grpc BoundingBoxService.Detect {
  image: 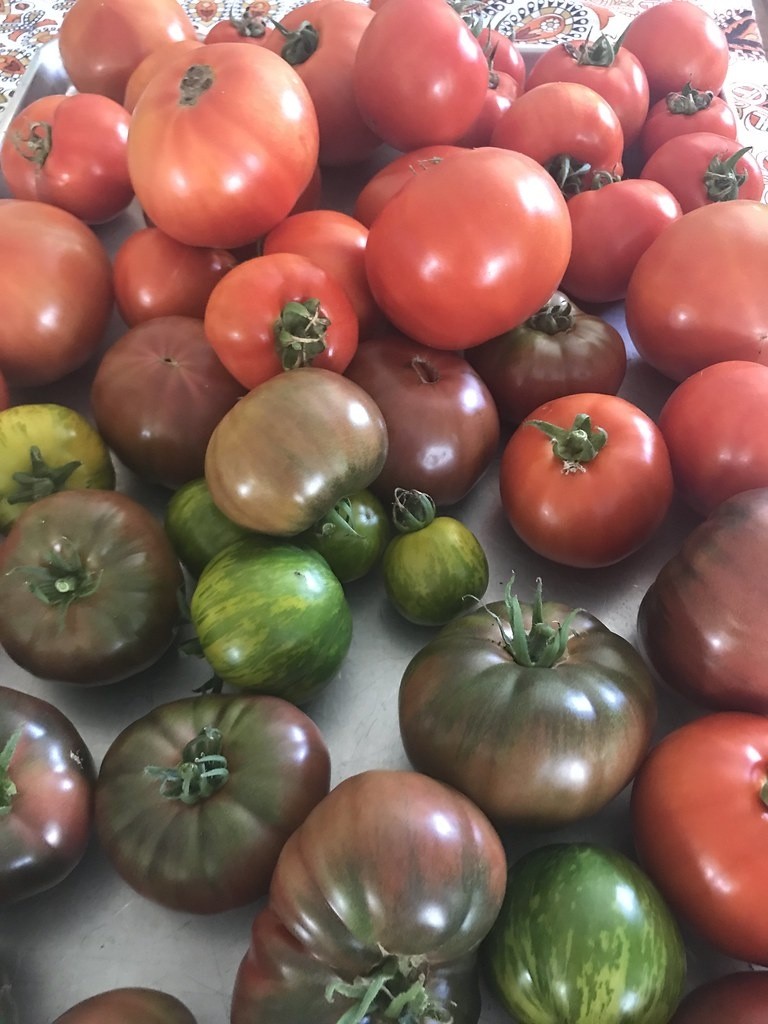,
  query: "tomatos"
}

[0,0,768,1024]
